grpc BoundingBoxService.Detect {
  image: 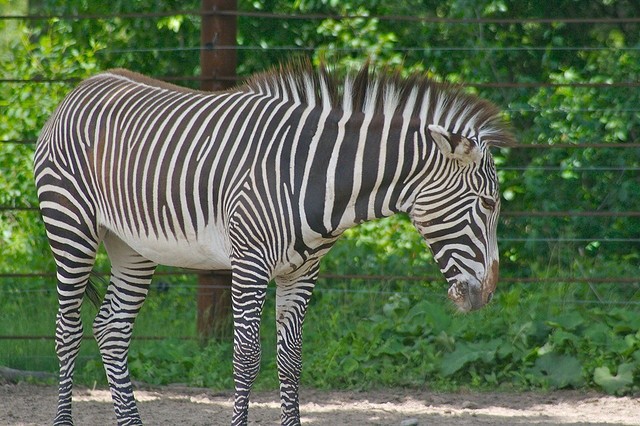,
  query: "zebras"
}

[34,56,519,426]
